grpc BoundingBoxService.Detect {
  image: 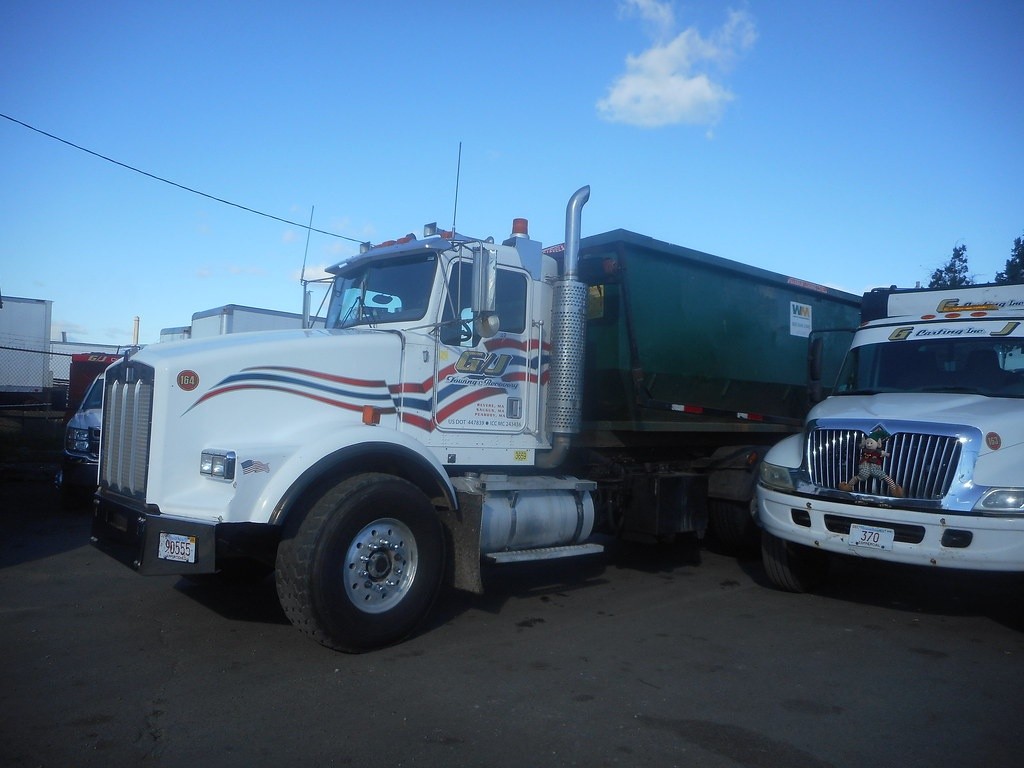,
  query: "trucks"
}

[61,372,128,491]
[94,184,865,656]
[741,282,1024,619]
